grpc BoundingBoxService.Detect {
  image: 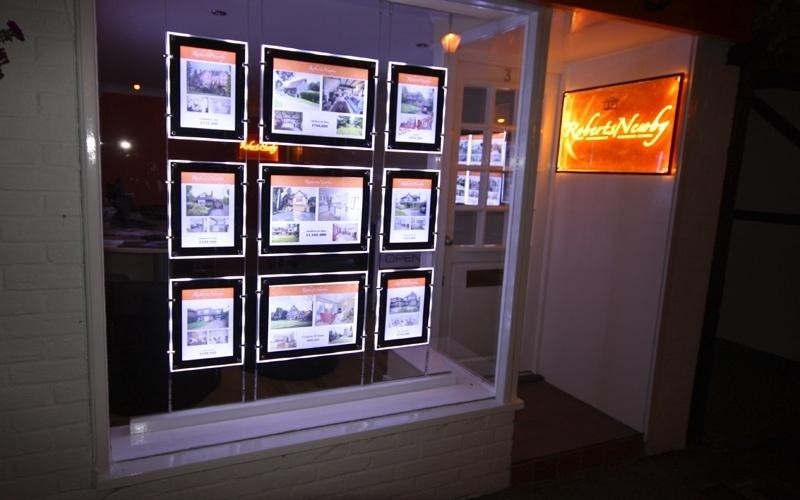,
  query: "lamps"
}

[441,12,461,53]
